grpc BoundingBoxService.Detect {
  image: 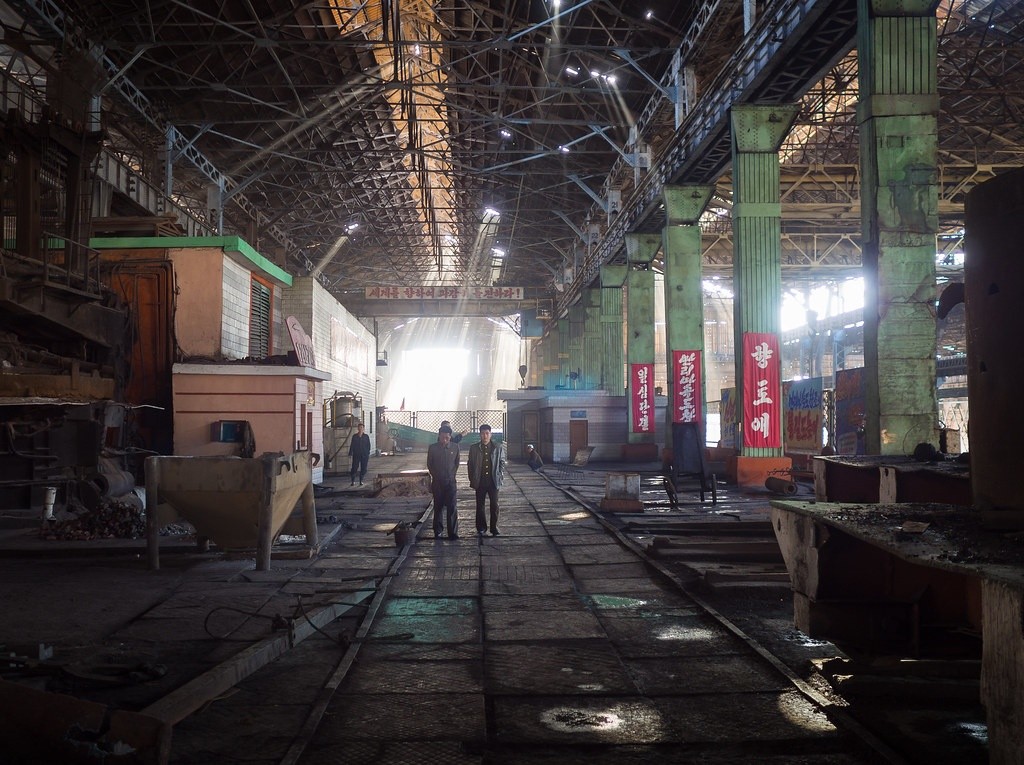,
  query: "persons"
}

[468,425,504,537]
[526,444,543,473]
[426,426,462,540]
[348,423,371,486]
[436,420,469,445]
